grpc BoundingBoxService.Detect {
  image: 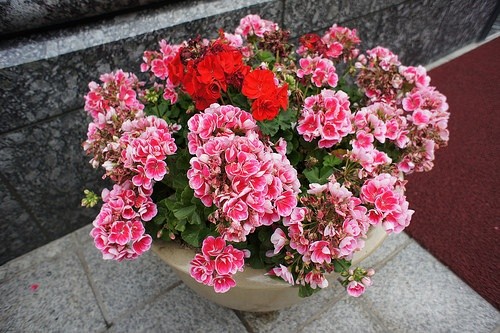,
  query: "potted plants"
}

[77,11,451,311]
